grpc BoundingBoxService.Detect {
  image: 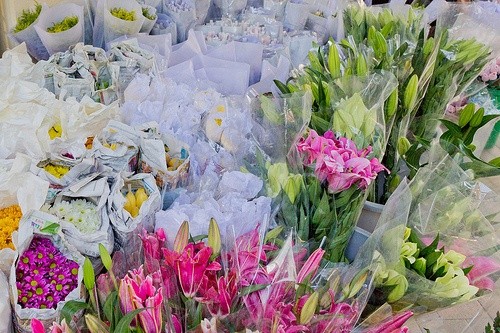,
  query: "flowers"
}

[0,0,500,333]
